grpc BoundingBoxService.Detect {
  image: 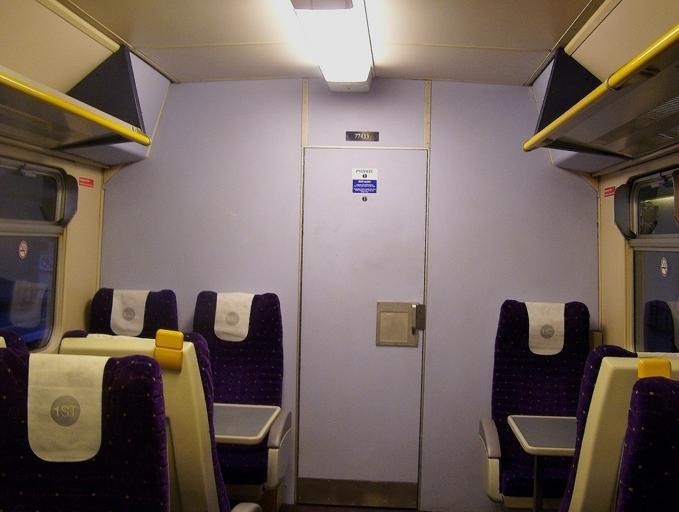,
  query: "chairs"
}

[477,298,676,511]
[0,286,293,511]
